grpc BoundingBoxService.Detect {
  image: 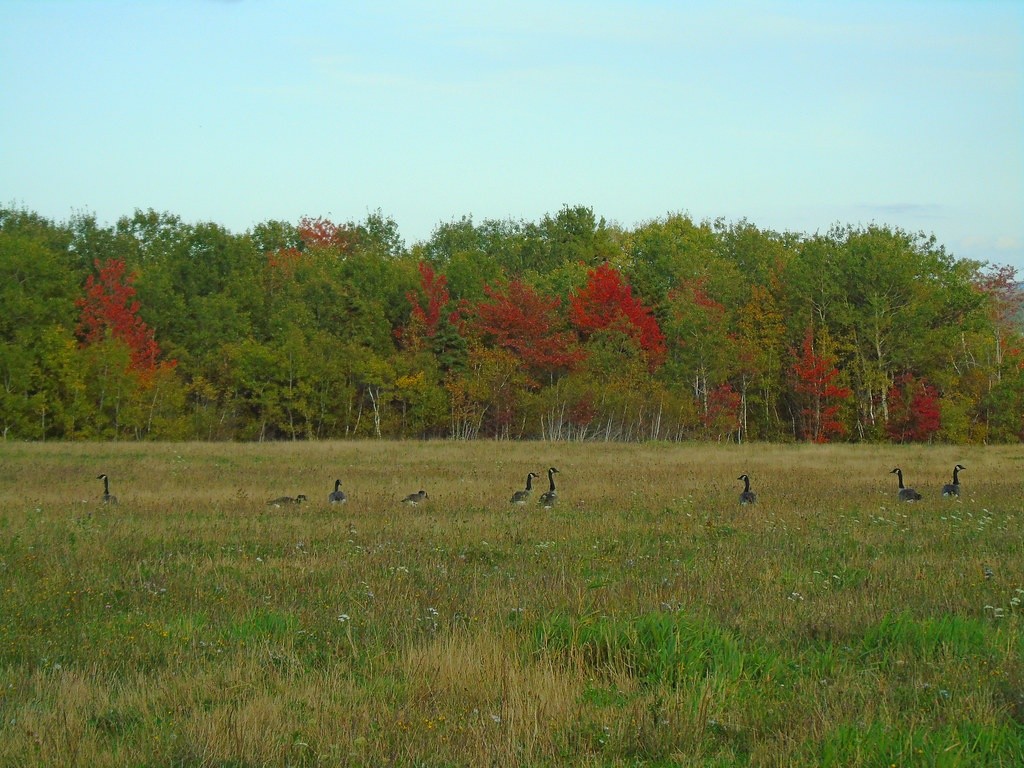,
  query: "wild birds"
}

[95,464,968,511]
